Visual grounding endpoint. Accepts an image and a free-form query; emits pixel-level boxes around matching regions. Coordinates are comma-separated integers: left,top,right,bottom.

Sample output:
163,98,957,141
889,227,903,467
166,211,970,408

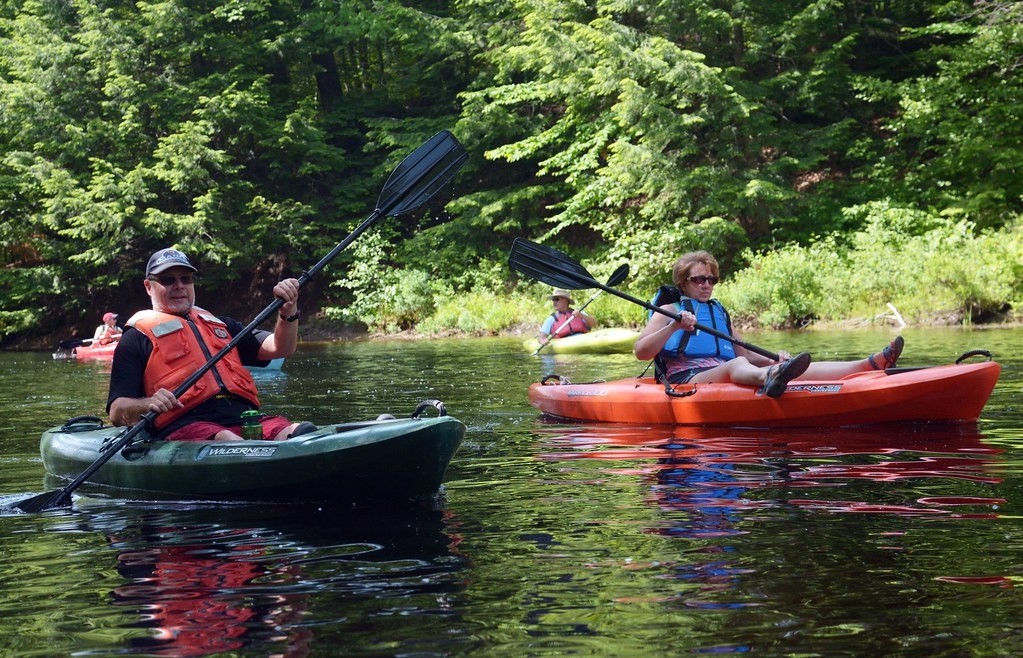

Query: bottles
240,410,263,440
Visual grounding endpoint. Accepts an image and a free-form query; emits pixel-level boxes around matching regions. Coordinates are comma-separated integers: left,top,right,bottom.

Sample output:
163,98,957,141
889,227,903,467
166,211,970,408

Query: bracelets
768,357,772,364
279,310,300,322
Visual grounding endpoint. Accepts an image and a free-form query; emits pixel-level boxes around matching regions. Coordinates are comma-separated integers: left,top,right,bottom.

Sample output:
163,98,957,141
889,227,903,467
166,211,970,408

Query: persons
92,313,122,348
538,288,597,346
635,250,904,398
106,249,396,441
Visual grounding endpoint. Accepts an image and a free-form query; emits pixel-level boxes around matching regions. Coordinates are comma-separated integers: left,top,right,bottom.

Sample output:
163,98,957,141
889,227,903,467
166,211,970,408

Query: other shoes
376,413,396,420
291,421,317,436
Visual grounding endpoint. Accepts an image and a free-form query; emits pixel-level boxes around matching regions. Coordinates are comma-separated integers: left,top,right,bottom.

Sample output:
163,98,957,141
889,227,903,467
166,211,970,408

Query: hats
103,313,118,322
547,290,574,305
146,249,197,277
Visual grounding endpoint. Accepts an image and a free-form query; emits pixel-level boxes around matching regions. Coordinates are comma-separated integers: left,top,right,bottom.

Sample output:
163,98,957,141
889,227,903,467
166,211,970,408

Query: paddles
9,129,471,509
59,333,122,350
529,262,631,356
506,235,779,362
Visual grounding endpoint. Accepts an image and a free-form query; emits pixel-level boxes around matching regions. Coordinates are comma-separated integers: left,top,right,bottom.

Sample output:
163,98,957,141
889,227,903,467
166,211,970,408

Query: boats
529,348,1002,441
38,400,470,504
73,340,124,361
523,325,642,355
244,357,286,373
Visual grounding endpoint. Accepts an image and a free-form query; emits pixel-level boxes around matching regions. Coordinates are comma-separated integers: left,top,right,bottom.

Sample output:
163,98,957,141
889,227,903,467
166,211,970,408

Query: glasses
552,297,567,301
147,275,195,285
685,276,718,284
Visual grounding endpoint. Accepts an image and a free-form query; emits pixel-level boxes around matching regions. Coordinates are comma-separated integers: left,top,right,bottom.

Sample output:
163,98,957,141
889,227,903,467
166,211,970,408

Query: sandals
869,336,903,370
764,353,810,398
